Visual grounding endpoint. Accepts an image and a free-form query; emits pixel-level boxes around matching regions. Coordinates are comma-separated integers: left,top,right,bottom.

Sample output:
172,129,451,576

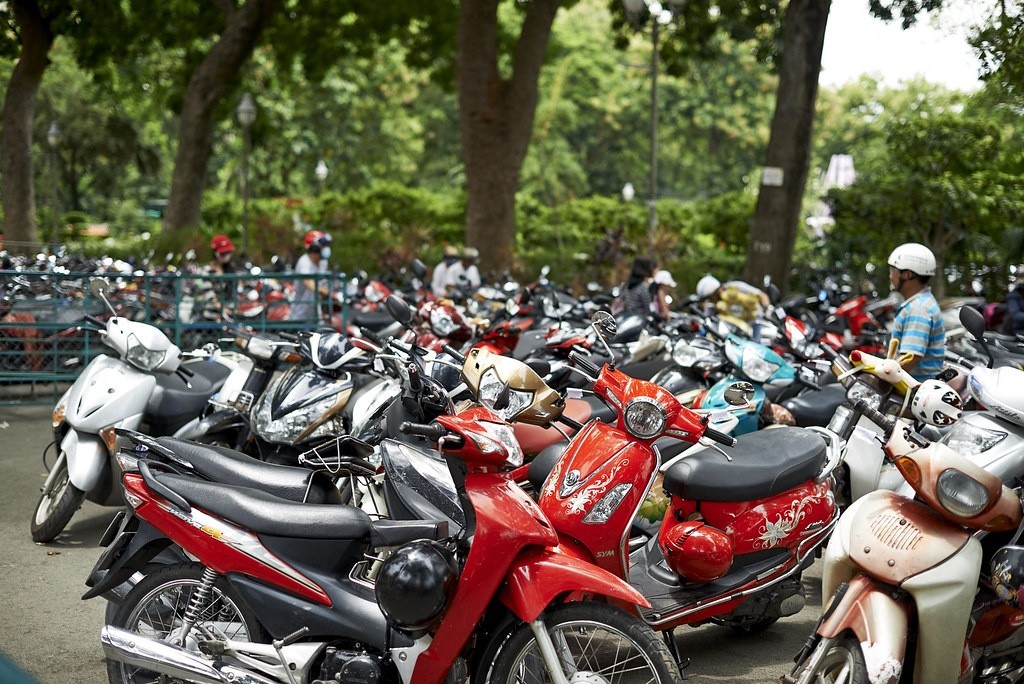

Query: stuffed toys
851,337,920,395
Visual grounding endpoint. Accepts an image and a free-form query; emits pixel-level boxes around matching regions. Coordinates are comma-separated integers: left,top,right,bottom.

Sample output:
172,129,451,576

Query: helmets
304,230,332,251
663,520,733,584
461,247,478,261
444,246,459,259
374,539,457,639
696,276,721,302
887,243,936,277
965,545,1024,648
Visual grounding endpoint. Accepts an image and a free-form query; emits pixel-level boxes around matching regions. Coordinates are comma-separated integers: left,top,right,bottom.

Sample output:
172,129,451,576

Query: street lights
235,91,258,245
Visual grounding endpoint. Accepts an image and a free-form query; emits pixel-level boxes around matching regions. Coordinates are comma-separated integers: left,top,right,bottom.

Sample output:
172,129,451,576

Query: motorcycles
0,243,1024,684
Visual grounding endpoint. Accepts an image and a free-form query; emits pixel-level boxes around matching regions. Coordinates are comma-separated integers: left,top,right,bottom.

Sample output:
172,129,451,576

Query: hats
211,235,235,254
655,270,677,288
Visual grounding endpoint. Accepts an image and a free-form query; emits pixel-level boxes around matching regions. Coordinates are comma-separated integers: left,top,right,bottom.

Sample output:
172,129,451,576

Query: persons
430,246,482,299
1000,281,1024,336
288,228,351,322
201,234,235,314
887,242,946,383
611,257,669,318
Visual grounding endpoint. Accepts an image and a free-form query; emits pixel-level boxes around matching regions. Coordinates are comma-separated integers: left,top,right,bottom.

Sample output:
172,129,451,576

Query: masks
218,253,232,263
321,247,331,259
